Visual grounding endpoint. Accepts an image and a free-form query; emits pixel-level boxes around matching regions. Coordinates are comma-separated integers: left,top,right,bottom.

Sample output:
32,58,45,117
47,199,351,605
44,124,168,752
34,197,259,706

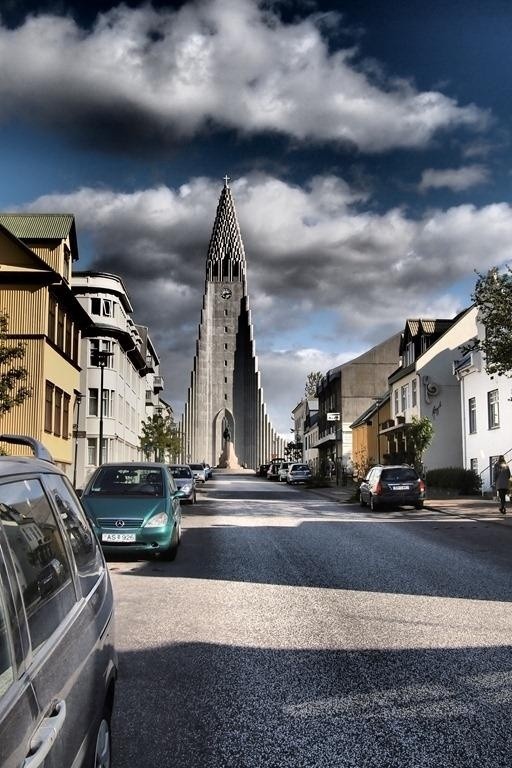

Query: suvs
356,461,427,513
0,430,122,768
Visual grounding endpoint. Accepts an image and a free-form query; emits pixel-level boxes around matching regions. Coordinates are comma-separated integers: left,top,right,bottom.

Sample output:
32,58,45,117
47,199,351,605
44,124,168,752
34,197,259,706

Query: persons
492,454,512,514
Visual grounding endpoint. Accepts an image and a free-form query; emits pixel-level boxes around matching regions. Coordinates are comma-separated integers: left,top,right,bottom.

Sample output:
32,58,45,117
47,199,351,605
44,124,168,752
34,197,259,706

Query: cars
73,459,219,564
259,456,312,485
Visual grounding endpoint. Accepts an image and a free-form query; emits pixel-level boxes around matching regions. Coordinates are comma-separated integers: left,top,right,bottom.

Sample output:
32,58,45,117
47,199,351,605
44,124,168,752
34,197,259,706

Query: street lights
371,394,384,468
89,348,115,470
